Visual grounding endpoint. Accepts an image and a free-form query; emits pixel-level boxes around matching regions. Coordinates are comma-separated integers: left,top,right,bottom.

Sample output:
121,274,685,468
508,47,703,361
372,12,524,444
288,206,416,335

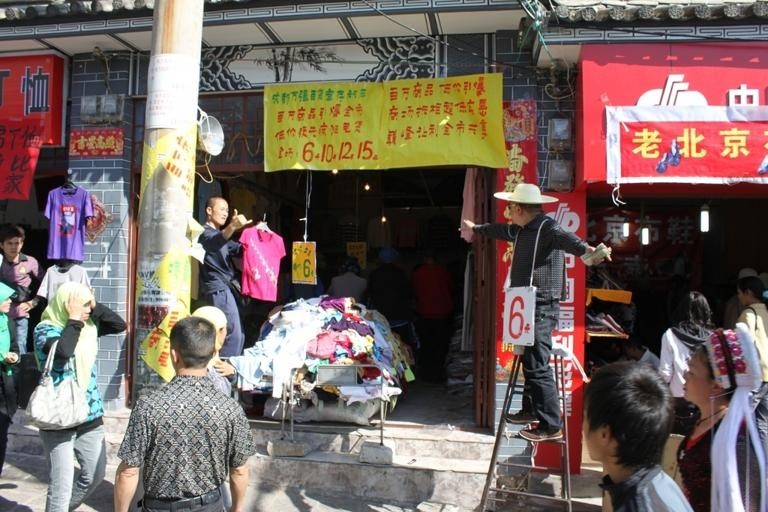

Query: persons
193,305,238,402
325,260,371,305
24,281,128,511
1,222,47,407
583,267,766,510
1,281,20,477
367,246,410,334
461,184,613,441
112,315,255,512
198,197,254,398
409,248,457,386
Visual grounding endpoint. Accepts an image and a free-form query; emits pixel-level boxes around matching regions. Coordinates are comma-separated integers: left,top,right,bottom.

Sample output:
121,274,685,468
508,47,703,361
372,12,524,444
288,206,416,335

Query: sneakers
519,430,563,442
507,412,540,423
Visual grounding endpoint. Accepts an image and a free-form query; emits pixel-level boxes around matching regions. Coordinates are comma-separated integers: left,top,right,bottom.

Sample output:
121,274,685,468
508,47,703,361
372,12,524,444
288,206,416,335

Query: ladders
479,344,570,512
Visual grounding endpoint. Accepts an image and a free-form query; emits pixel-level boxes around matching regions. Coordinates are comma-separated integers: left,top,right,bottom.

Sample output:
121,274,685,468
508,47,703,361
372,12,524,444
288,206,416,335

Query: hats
494,184,559,205
707,322,762,389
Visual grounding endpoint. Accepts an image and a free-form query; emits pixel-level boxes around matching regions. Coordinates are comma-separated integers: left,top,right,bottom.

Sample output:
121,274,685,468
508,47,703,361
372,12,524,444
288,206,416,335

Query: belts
138,489,220,509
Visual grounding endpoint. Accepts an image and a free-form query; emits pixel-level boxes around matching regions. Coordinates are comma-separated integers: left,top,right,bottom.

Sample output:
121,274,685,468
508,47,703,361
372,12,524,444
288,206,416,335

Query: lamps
622,200,710,246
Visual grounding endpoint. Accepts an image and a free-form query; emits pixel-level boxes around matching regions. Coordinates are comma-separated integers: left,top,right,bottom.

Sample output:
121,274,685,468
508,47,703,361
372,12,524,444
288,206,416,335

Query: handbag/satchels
669,397,701,435
8,284,32,302
25,340,89,430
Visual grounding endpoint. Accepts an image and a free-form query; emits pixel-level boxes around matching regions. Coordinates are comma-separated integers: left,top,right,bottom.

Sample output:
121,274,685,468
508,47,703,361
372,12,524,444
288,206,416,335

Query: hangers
59,173,78,196
255,212,273,235
53,258,74,271
